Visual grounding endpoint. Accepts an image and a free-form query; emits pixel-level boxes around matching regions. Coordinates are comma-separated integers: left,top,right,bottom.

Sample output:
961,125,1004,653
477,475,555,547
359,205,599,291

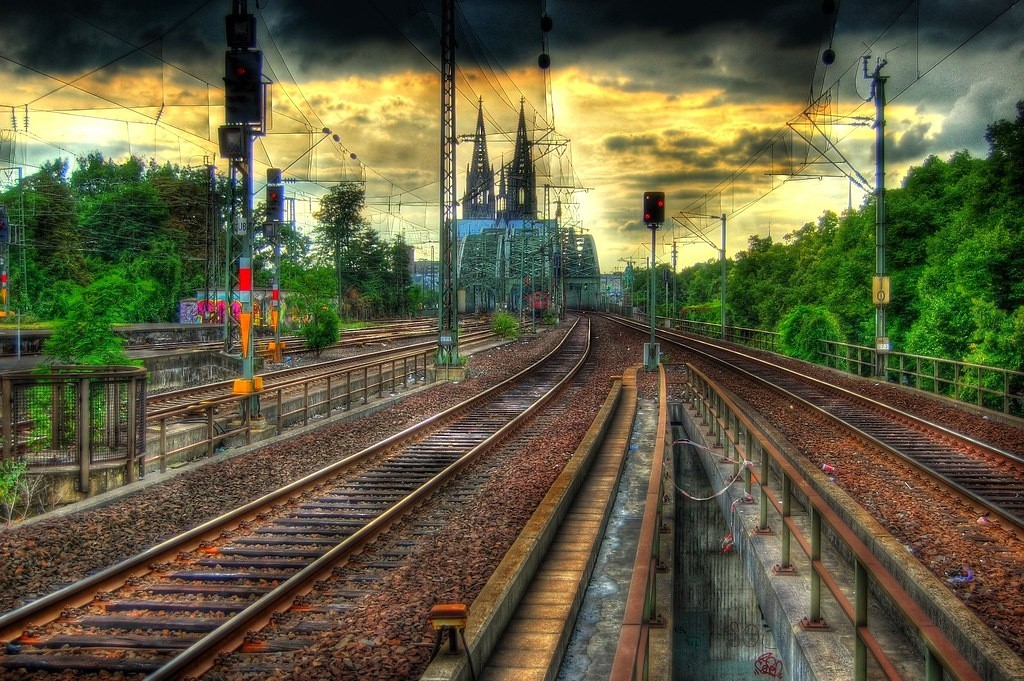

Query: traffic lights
643,191,665,223
263,222,276,237
266,184,285,222
0,217,9,241
218,126,245,156
225,48,265,128
